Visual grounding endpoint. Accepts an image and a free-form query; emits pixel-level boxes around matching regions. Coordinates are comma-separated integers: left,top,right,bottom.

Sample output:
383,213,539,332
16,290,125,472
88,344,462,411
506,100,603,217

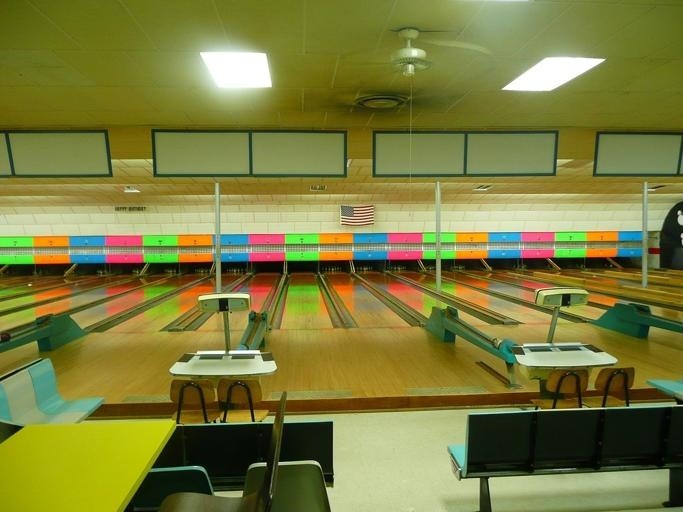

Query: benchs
447,405,683,511
174,421,335,489
0,357,104,426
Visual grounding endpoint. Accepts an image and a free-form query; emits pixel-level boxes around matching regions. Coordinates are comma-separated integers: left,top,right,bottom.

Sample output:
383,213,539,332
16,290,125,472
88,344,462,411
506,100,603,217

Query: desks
646,374,683,403
0,417,175,512
168,353,277,378
512,342,618,368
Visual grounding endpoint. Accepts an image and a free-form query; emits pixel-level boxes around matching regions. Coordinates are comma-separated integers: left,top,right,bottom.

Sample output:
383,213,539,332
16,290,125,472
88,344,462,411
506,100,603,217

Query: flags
340,205,374,226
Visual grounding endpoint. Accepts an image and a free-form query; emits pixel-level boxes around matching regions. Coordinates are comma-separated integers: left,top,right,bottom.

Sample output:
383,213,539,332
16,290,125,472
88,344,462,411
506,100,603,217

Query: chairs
242,460,332,511
217,379,269,421
531,369,588,407
595,369,634,407
134,465,213,511
170,379,222,423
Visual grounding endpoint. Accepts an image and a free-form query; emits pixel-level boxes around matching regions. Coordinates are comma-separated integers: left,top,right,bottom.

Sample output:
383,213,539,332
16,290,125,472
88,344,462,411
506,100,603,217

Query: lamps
401,65,418,77
354,95,407,111
